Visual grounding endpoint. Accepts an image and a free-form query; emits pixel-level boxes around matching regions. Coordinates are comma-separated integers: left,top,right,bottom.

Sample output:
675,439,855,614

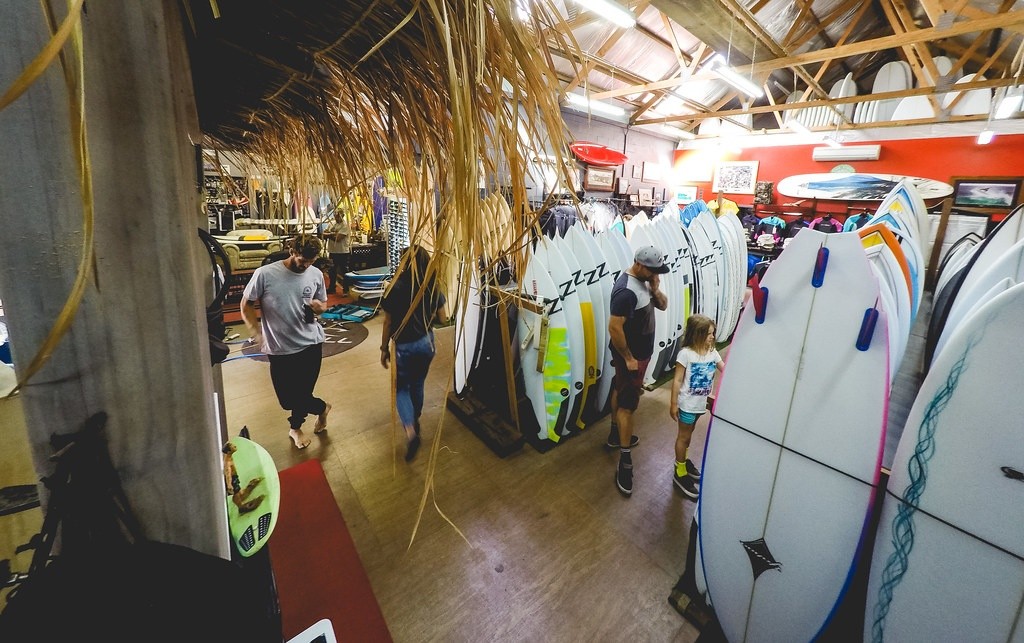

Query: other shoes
343,289,350,297
405,436,421,462
415,423,419,435
327,287,336,294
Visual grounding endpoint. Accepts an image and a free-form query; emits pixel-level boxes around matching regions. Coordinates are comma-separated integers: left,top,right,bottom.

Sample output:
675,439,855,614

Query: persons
608,245,670,495
380,245,447,462
240,234,332,450
322,208,350,298
670,315,726,499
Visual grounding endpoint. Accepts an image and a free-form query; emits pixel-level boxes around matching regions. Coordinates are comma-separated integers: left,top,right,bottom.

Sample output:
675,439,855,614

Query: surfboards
347,165,755,448
691,175,1024,643
224,436,282,557
254,166,404,237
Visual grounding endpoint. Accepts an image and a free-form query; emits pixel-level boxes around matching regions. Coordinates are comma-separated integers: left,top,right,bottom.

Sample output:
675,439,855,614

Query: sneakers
673,462,699,499
616,457,633,495
685,448,701,480
609,433,639,448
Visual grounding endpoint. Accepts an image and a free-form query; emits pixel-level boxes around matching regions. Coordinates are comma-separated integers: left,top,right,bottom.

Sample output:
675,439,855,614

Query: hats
634,245,670,273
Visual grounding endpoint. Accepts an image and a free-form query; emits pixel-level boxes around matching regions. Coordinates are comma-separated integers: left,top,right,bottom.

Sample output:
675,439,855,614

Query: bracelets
380,345,389,351
